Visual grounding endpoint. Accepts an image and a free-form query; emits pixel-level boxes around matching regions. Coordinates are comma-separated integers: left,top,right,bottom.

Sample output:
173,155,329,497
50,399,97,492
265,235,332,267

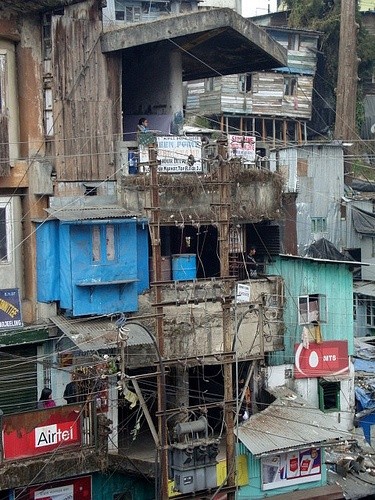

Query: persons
63,370,88,404
38,388,56,409
138,118,162,134
245,246,258,279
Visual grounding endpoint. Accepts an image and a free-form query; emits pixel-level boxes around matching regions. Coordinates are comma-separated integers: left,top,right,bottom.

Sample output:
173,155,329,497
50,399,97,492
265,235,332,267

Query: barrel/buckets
171,254,196,281
148,256,170,282
